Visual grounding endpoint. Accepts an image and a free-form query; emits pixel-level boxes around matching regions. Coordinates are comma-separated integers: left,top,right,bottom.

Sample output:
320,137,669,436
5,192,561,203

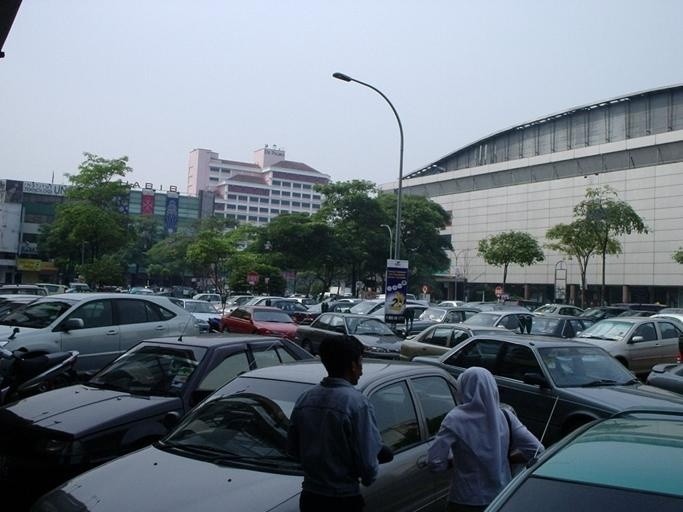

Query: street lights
333,70,408,293
379,219,405,259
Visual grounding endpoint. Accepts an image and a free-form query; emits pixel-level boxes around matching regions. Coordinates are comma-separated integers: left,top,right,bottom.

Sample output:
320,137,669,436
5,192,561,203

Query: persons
427,366,546,512
287,335,382,512
